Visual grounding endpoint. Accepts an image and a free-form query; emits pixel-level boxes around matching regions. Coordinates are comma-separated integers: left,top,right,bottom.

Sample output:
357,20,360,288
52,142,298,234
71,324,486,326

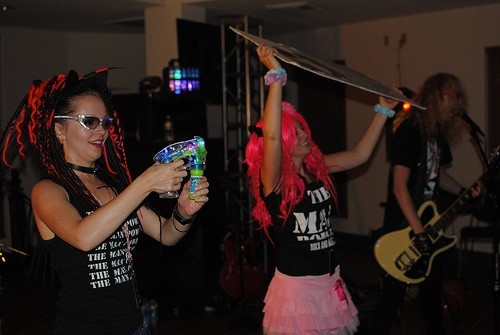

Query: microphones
457,109,485,136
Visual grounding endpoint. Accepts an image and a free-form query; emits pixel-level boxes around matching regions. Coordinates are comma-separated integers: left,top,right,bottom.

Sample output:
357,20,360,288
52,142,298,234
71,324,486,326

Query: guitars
373,148,500,284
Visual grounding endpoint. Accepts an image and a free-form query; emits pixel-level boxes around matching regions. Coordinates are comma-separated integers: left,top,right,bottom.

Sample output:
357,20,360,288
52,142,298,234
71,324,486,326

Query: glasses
54,115,114,130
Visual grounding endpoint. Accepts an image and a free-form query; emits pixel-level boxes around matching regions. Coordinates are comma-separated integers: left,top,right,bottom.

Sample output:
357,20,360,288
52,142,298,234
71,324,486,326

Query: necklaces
67,162,98,174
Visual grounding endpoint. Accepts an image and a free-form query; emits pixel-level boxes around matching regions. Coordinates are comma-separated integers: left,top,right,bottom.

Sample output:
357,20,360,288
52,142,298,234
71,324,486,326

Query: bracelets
173,204,196,233
263,68,287,86
373,104,395,118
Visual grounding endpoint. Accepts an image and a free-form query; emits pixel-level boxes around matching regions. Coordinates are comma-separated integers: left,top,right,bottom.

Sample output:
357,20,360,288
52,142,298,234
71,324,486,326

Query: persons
240,42,403,335
0,67,209,335
392,72,490,335
